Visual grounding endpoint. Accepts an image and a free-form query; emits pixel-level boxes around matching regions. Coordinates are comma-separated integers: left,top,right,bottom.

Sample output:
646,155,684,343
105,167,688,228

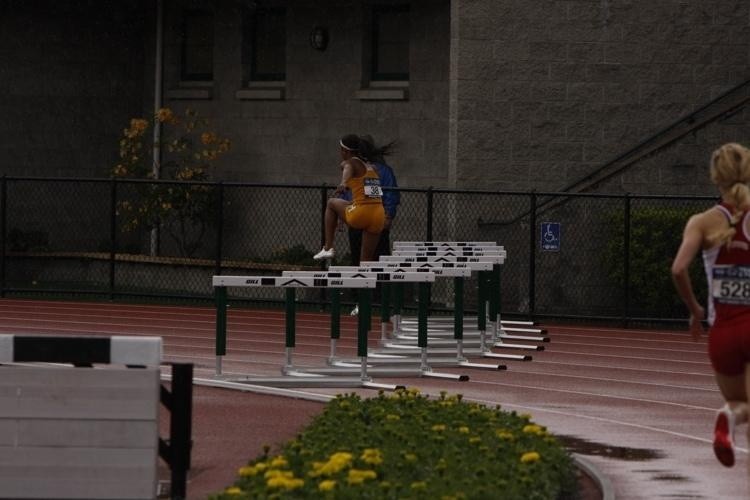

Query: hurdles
392,242,548,336
0,332,193,499
391,251,551,342
327,265,508,371
213,275,406,390
282,269,471,381
359,261,534,361
378,254,542,353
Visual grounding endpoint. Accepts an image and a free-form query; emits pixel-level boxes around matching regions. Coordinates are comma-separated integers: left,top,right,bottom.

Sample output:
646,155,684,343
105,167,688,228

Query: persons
671,142,750,467
312,133,387,318
344,135,401,316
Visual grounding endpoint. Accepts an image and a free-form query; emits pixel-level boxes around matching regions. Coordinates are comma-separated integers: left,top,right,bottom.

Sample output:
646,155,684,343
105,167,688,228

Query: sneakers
351,305,359,317
712,411,736,467
312,247,336,261
